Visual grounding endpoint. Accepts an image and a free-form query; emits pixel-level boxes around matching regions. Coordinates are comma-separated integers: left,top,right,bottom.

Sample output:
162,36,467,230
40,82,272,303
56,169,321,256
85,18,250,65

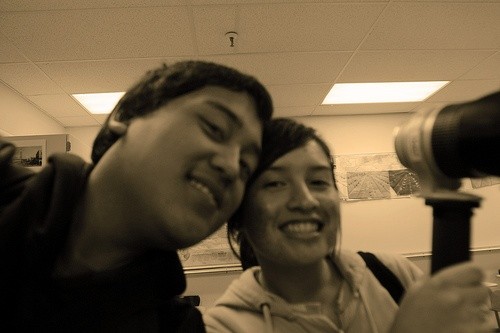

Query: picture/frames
177,221,243,274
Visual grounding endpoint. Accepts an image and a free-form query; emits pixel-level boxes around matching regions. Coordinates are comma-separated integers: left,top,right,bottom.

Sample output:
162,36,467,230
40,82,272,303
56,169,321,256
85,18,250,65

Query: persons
201,117,490,333
0,60,273,333
